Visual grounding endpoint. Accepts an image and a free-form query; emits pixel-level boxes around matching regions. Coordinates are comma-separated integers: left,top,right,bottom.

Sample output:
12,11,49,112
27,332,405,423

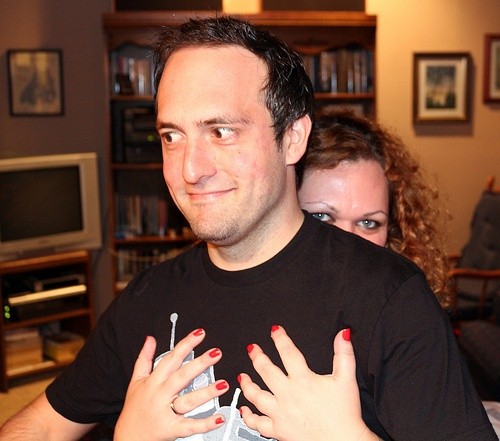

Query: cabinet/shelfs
104,10,378,294
0,249,96,393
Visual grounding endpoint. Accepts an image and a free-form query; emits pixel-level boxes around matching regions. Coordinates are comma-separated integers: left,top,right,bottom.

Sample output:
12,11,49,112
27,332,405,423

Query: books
117,247,164,280
113,194,169,236
303,45,371,95
108,52,156,97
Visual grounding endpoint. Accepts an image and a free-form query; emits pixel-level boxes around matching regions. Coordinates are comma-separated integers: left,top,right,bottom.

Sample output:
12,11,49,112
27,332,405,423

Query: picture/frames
483,32,500,102
413,50,471,122
9,47,63,116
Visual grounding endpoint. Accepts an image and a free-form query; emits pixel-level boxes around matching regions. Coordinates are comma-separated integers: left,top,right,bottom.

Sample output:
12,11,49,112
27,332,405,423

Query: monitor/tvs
0,152,102,262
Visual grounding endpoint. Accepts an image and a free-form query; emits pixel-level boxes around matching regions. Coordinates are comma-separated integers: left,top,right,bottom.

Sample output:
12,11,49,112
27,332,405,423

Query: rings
171,397,184,416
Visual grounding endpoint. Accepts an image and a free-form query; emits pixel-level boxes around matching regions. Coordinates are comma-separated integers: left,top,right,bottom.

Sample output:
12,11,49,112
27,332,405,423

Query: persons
113,110,458,441
0,10,500,441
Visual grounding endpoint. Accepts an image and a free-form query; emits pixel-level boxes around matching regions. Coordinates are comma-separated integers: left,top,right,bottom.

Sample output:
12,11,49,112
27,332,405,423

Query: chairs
442,175,500,388
